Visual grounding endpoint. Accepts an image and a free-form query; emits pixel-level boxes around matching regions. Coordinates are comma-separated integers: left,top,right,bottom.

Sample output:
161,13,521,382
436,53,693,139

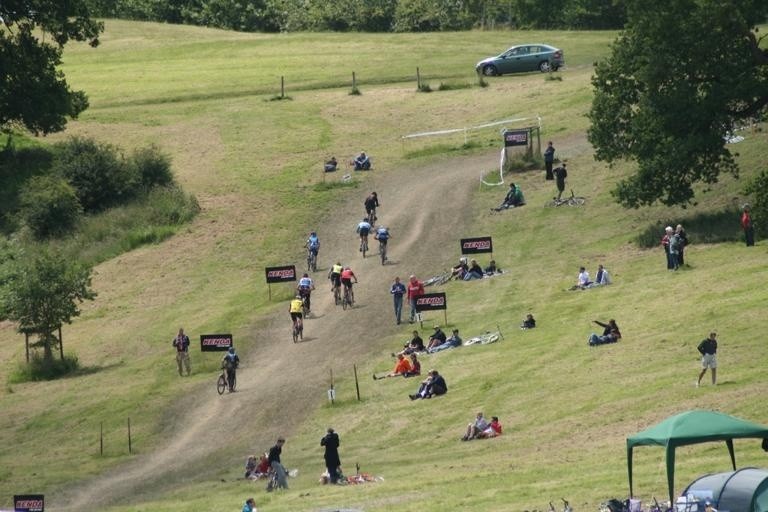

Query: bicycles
290,308,304,343
215,364,238,398
372,234,389,265
341,281,358,312
358,229,372,259
301,245,317,273
327,276,342,306
548,188,586,207
422,266,456,289
294,287,313,317
364,205,380,227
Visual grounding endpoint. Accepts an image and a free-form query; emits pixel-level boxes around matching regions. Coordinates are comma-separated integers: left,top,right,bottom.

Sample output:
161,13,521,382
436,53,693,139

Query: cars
471,43,566,79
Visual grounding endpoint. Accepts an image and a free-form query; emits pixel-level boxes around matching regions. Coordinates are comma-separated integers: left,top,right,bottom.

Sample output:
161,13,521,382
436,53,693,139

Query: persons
321,427,341,485
741,203,754,247
172,328,191,377
696,332,717,388
222,347,240,392
269,437,289,489
242,498,256,512
